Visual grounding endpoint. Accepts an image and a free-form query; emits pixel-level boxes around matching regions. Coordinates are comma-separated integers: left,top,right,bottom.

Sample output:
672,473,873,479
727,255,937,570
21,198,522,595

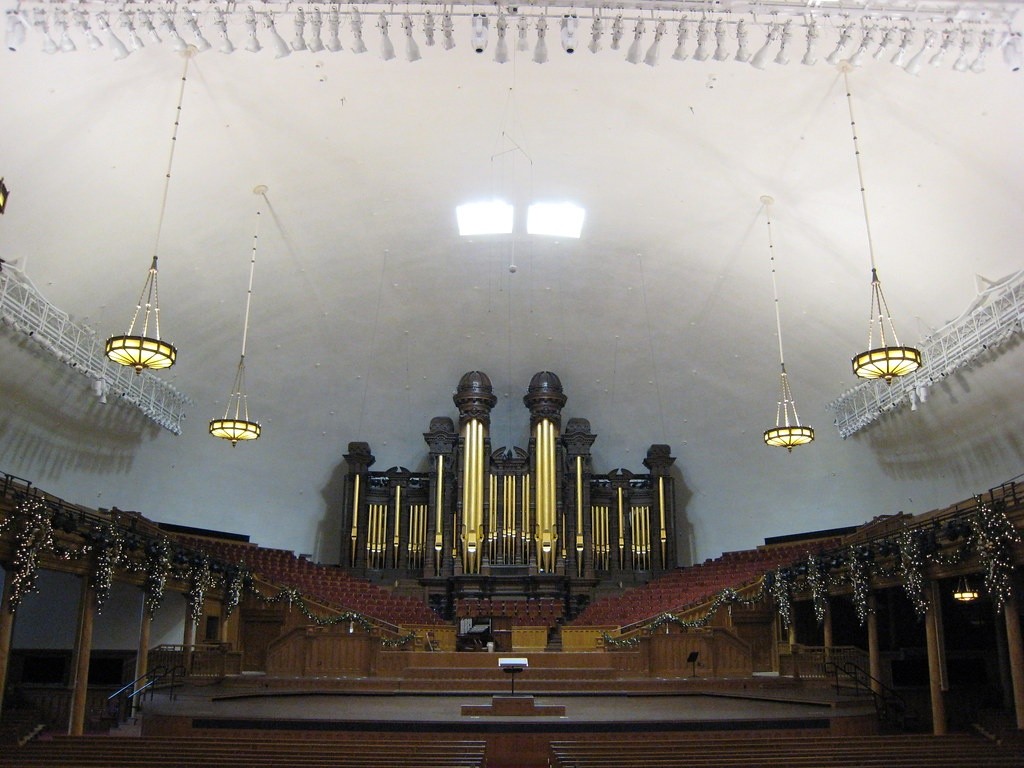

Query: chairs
492,600,502,608
540,599,562,607
529,607,539,616
570,537,842,629
517,599,527,607
480,609,490,616
505,600,514,606
493,608,502,616
456,600,466,608
505,607,515,616
468,600,478,608
512,616,556,627
175,535,445,626
470,608,478,617
529,598,539,607
541,605,550,616
480,600,490,608
517,607,527,616
552,606,562,617
456,607,467,617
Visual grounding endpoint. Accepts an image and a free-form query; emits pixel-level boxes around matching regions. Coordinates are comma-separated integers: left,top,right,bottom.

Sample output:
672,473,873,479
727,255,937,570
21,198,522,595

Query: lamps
953,577,979,602
516,16,530,52
493,12,512,64
761,195,813,452
625,4,1024,76
532,14,551,64
834,61,922,386
558,7,581,54
825,315,1024,439
610,8,625,50
8,6,367,62
103,45,198,376
587,8,604,54
400,11,422,62
0,307,195,437
374,10,396,61
423,10,437,46
440,5,457,50
209,185,268,445
471,12,490,54
